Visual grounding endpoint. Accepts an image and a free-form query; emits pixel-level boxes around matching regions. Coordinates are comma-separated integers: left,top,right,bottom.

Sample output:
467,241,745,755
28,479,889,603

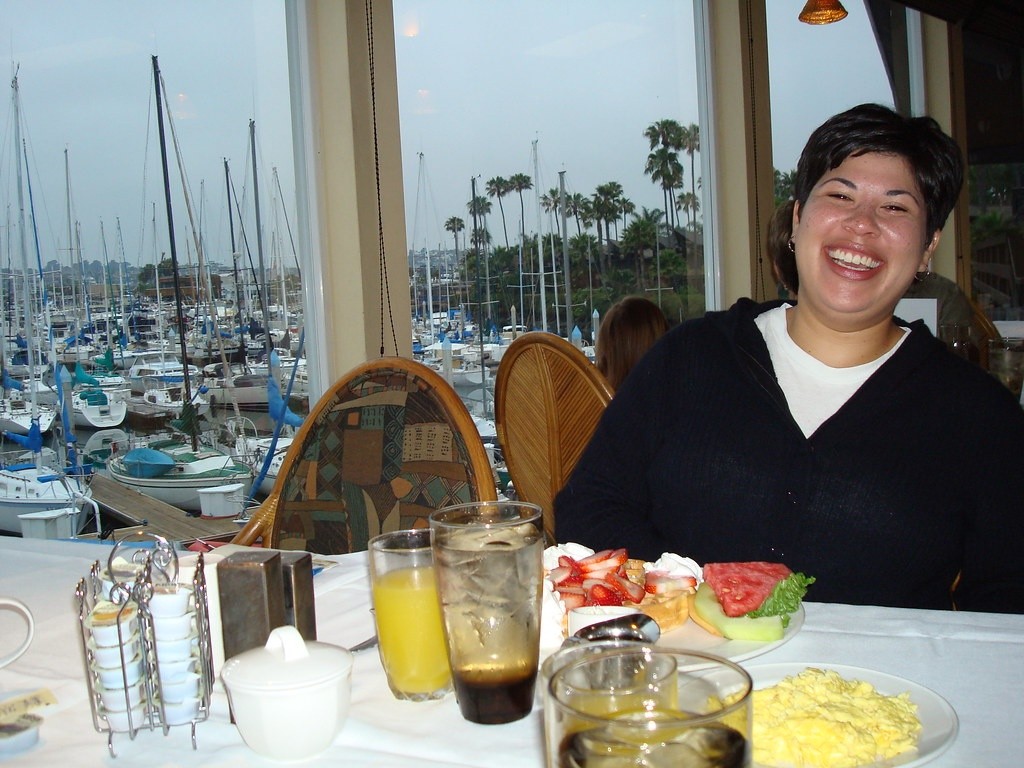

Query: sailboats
0,75,92,534
1,122,595,497
108,55,260,509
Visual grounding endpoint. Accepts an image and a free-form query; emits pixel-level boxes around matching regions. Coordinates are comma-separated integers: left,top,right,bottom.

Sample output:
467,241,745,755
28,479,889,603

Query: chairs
493,331,615,548
228,355,499,550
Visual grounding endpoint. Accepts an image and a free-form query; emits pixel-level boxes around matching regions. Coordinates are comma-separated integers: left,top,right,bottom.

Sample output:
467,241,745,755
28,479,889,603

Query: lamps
798,0,849,26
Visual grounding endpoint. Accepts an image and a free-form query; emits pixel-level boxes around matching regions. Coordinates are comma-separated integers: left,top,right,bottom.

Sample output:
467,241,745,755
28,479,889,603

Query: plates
540,585,804,675
737,664,960,767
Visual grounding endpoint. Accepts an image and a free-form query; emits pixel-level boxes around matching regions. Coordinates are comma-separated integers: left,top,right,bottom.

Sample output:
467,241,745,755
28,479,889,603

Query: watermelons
704,562,793,618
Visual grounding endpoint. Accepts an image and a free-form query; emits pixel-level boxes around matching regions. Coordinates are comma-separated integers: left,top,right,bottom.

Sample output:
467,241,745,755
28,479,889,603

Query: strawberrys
546,549,698,612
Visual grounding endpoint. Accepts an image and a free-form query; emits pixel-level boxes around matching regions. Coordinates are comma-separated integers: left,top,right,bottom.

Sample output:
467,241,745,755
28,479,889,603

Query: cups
429,501,543,724
541,640,753,768
987,337,1024,403
568,605,639,642
939,319,980,368
217,626,353,757
366,531,454,702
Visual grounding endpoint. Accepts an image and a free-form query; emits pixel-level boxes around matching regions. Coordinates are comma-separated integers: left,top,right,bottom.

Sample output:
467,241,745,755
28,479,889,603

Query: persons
595,297,671,393
768,197,800,299
530,103,1024,615
903,271,977,349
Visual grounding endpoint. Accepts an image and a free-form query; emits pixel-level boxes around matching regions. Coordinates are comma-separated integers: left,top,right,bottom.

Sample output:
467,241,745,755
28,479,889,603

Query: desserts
537,580,566,672
645,552,703,582
542,542,595,570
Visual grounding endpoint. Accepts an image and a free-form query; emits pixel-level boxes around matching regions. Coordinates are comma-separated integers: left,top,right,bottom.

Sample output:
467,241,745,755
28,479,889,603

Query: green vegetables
748,573,816,628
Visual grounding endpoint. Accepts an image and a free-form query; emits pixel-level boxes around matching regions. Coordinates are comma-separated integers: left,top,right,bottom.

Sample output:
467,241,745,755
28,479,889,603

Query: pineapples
690,584,784,639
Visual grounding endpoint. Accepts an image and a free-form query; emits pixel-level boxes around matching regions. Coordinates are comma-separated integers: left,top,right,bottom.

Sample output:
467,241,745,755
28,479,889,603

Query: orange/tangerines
623,591,689,633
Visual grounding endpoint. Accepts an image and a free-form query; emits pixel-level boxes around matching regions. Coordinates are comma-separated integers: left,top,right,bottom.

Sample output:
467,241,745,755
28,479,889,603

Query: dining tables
0,535,1024,768
